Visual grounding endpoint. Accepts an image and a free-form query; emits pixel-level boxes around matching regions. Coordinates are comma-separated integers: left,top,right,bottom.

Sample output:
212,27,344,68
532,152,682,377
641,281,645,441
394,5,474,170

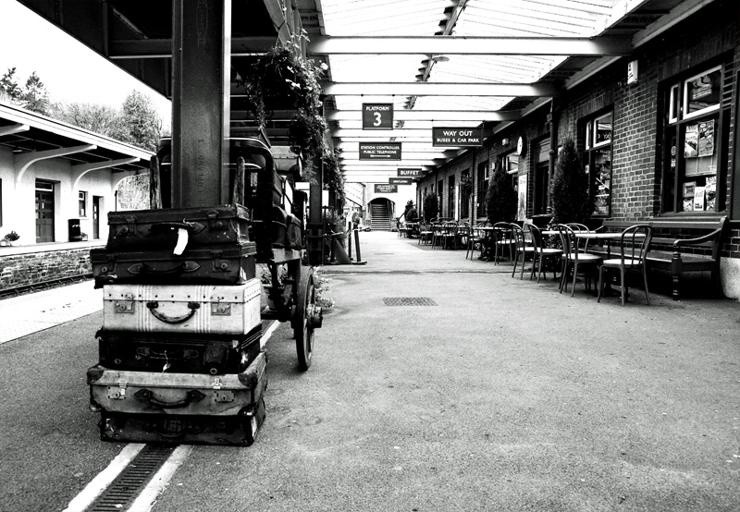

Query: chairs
395,215,653,306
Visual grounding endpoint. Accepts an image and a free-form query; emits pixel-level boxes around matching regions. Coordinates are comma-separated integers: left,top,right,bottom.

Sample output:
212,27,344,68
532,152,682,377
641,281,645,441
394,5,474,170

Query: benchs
597,209,729,302
